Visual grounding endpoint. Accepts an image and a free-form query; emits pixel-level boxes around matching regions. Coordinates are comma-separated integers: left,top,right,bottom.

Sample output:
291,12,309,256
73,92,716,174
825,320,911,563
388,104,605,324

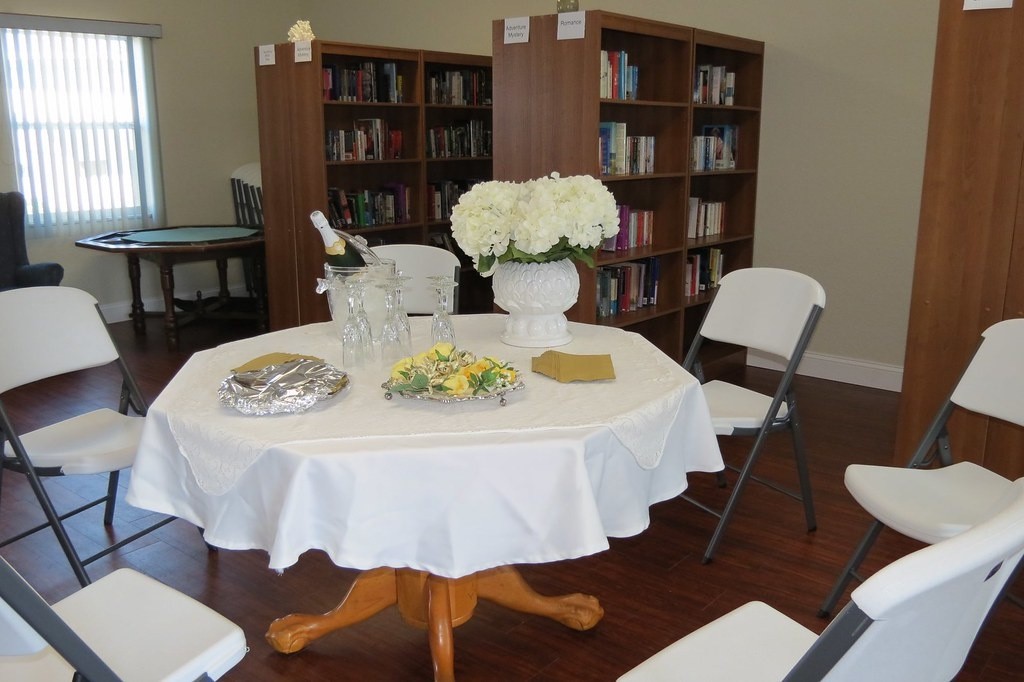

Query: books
429,115,493,158
692,123,738,171
327,182,411,229
688,195,725,238
685,247,724,296
596,257,660,318
597,121,656,175
430,178,477,220
323,58,403,104
599,47,637,101
325,117,403,161
600,203,654,251
429,231,454,256
694,62,736,106
430,67,492,105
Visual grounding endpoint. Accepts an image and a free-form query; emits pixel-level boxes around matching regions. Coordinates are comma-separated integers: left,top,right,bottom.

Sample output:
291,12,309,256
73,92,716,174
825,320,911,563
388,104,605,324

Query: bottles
310,211,366,268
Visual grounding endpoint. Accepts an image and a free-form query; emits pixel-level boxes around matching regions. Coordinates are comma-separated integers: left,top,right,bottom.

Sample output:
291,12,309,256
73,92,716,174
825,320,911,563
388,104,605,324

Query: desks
74,224,269,351
124,314,726,682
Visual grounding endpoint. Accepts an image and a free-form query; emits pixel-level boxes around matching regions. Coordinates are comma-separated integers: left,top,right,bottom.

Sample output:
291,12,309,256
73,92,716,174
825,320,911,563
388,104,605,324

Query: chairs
0,191,64,292
616,476,1024,682
0,285,218,588
0,556,250,682
361,245,461,316
816,317,1024,621
644,266,826,564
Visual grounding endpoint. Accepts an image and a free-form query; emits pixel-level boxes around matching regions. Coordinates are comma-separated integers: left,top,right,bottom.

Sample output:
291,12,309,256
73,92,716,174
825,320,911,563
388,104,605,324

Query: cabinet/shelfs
252,40,494,332
491,9,766,386
894,0,1024,481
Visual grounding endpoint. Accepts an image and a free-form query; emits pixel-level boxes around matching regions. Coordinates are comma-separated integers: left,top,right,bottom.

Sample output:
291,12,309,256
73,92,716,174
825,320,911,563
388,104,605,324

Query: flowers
384,342,516,398
449,171,620,279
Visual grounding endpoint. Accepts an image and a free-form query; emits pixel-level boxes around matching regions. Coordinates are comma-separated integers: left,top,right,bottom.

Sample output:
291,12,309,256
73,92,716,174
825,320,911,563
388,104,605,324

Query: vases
491,257,581,349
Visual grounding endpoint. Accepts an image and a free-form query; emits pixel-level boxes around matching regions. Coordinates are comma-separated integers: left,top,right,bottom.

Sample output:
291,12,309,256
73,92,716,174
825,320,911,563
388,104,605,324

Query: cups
316,258,396,345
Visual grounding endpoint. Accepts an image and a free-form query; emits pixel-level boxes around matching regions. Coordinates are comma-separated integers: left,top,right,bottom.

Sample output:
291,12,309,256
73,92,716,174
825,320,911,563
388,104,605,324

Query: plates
381,377,524,407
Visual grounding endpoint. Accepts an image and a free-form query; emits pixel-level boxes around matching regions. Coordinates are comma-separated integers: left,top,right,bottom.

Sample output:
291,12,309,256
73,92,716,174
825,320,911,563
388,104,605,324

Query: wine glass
340,274,457,368
